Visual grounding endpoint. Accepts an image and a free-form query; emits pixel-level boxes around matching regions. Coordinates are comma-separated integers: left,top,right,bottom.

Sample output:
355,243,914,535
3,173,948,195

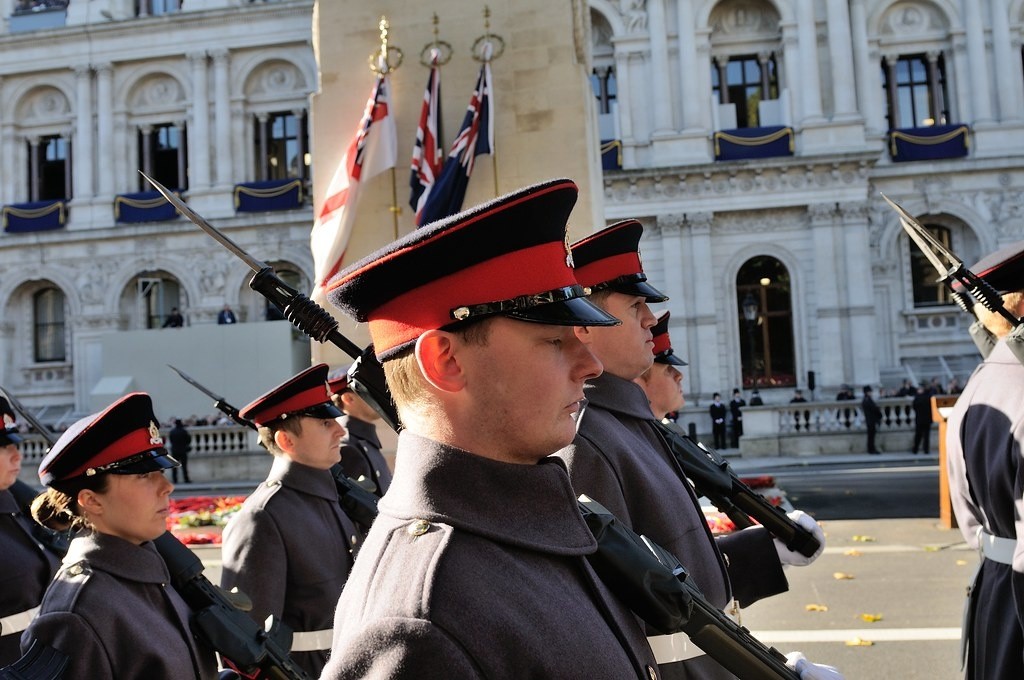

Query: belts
0,604,42,637
291,628,334,652
981,532,1017,565
646,596,742,664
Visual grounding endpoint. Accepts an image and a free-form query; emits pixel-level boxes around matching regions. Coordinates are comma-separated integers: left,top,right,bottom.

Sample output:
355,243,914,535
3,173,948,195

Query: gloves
772,510,825,567
716,418,723,424
784,651,844,680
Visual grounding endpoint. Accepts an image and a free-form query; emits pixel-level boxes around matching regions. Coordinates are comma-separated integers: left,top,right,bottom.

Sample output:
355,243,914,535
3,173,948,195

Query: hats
733,387,739,394
951,239,1024,304
238,363,347,429
840,383,850,390
649,309,688,366
752,389,760,394
38,393,182,487
863,385,873,393
324,178,624,364
919,379,929,386
326,365,354,395
569,218,670,304
713,392,720,399
0,396,25,447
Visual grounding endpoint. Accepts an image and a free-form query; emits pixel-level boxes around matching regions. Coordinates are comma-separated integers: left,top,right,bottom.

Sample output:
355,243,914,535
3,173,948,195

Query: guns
881,191,1024,328
688,477,758,530
166,362,382,518
899,215,979,322
135,168,801,680
0,385,316,680
655,419,820,558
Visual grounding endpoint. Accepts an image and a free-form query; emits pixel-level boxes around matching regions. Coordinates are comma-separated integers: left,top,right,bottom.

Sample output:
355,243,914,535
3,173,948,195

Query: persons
877,374,962,455
317,176,842,680
169,418,192,484
555,213,827,680
788,390,808,403
0,394,75,680
218,305,237,325
330,368,392,534
634,309,685,422
748,392,764,405
709,392,729,451
947,238,1024,680
218,362,365,680
162,309,184,328
861,386,883,457
834,385,859,429
19,392,249,680
730,388,747,449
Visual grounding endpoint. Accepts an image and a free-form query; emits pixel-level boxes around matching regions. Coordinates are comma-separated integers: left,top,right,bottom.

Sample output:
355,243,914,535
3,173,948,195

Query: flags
311,17,396,303
418,3,494,230
409,18,445,212
